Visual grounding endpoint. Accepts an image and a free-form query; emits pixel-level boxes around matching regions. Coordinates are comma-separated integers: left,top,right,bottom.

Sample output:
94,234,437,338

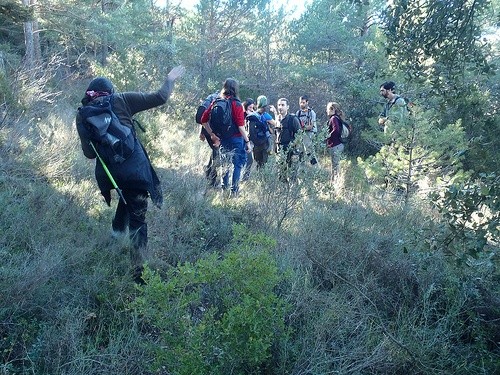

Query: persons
245,95,278,171
377,80,412,141
295,95,321,172
325,102,345,174
77,64,187,285
199,89,224,190
241,98,255,182
275,97,302,186
201,77,252,194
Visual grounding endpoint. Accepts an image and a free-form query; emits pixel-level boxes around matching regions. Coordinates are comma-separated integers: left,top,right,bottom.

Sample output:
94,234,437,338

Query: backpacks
196,93,220,124
209,96,240,139
331,114,353,143
246,113,272,146
76,94,137,166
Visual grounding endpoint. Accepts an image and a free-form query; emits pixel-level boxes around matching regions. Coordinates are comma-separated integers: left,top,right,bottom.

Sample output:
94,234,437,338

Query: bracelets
245,140,250,143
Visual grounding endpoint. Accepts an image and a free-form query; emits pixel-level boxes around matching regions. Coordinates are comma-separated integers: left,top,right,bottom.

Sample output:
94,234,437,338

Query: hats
257,95,267,107
